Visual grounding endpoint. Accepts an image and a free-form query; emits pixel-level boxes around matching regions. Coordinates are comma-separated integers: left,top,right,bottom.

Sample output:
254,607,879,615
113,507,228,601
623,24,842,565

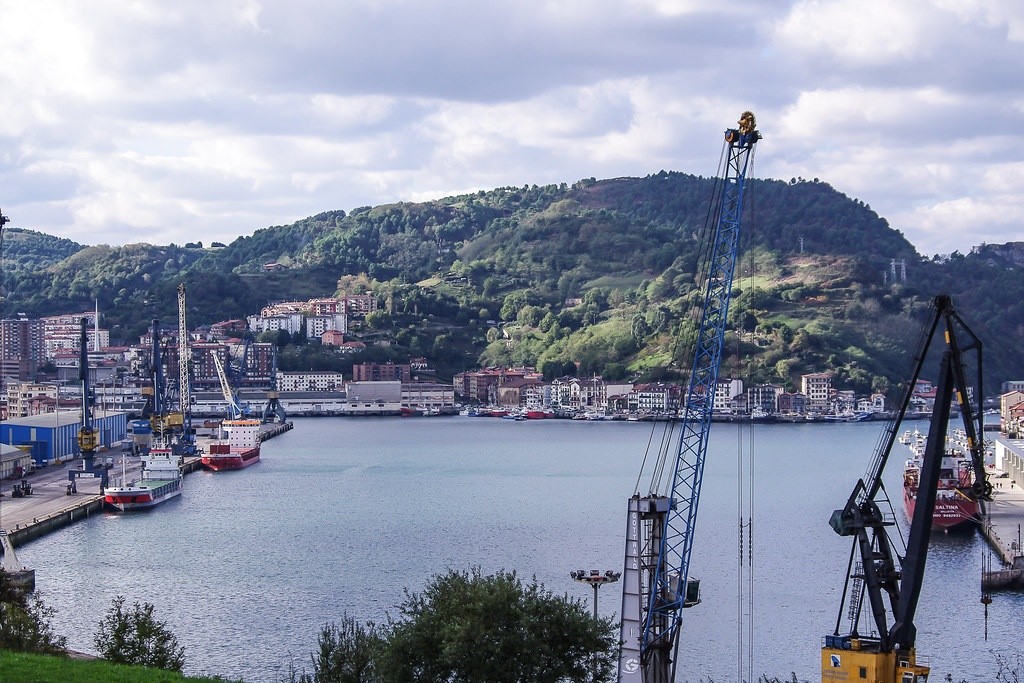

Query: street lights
569,569,622,683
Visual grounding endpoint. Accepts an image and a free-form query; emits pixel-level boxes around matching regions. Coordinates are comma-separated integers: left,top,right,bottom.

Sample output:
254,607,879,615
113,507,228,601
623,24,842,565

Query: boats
625,414,639,421
896,423,982,533
200,425,261,472
104,420,184,512
484,393,614,420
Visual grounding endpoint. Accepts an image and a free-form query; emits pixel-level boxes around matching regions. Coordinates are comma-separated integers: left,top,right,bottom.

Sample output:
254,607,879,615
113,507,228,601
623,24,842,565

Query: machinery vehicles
815,291,999,681
614,110,764,683
211,352,262,427
65,317,114,498
147,283,199,458
